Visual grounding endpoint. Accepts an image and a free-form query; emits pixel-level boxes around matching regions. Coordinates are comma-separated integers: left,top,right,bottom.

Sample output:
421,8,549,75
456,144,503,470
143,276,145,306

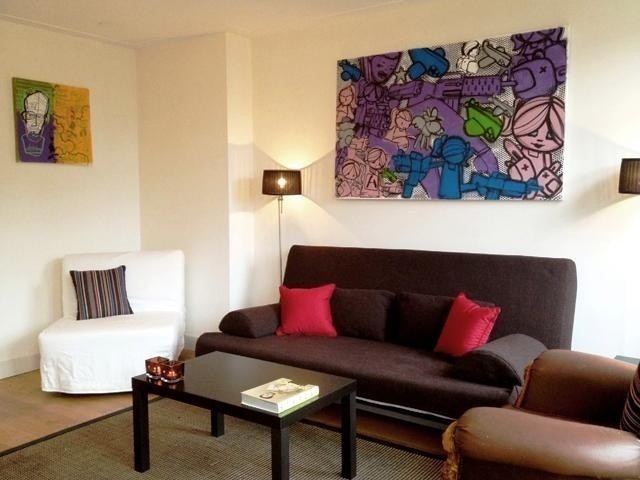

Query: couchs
196,245,577,431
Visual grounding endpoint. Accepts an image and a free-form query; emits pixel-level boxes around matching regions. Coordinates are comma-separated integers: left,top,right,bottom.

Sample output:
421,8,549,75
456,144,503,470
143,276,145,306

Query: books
239,376,321,415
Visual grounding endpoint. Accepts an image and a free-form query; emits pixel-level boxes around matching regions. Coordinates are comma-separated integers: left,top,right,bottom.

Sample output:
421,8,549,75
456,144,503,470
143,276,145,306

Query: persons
258,379,310,400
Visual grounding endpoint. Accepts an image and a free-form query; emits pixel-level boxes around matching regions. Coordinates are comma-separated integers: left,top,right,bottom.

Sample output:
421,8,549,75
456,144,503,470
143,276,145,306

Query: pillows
70,265,134,320
618,363,640,439
276,285,501,358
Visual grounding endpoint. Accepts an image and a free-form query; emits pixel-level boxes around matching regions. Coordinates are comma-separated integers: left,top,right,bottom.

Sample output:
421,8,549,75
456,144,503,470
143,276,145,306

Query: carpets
0,396,448,480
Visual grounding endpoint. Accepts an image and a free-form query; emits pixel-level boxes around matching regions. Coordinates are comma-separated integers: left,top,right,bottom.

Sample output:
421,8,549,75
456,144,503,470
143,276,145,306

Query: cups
145,355,185,385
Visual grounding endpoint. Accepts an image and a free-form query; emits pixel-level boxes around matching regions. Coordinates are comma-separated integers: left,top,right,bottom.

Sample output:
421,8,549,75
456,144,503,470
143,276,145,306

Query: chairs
38,249,186,395
442,349,640,480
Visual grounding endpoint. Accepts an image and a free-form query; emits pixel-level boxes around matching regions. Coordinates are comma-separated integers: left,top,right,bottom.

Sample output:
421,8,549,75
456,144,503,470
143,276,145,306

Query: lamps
619,159,640,193
262,170,301,284
